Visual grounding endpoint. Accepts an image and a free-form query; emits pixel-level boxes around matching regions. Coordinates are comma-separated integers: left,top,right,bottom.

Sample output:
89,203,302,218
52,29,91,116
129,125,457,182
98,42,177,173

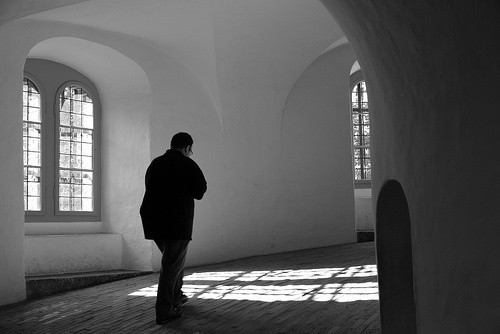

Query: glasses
189,148,193,155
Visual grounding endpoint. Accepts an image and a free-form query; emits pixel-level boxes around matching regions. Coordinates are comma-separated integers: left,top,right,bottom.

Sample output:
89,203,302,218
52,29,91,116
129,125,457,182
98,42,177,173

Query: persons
140,132,209,325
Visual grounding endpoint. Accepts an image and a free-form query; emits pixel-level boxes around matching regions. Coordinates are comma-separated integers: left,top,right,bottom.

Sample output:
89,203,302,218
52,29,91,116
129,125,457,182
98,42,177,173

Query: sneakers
157,308,182,325
174,295,188,307
